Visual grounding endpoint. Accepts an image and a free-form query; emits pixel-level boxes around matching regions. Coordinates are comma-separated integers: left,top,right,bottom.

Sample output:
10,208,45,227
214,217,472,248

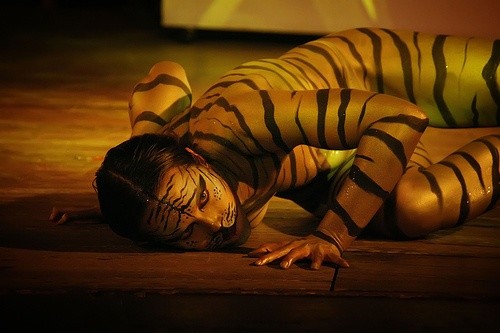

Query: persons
48,28,500,272
